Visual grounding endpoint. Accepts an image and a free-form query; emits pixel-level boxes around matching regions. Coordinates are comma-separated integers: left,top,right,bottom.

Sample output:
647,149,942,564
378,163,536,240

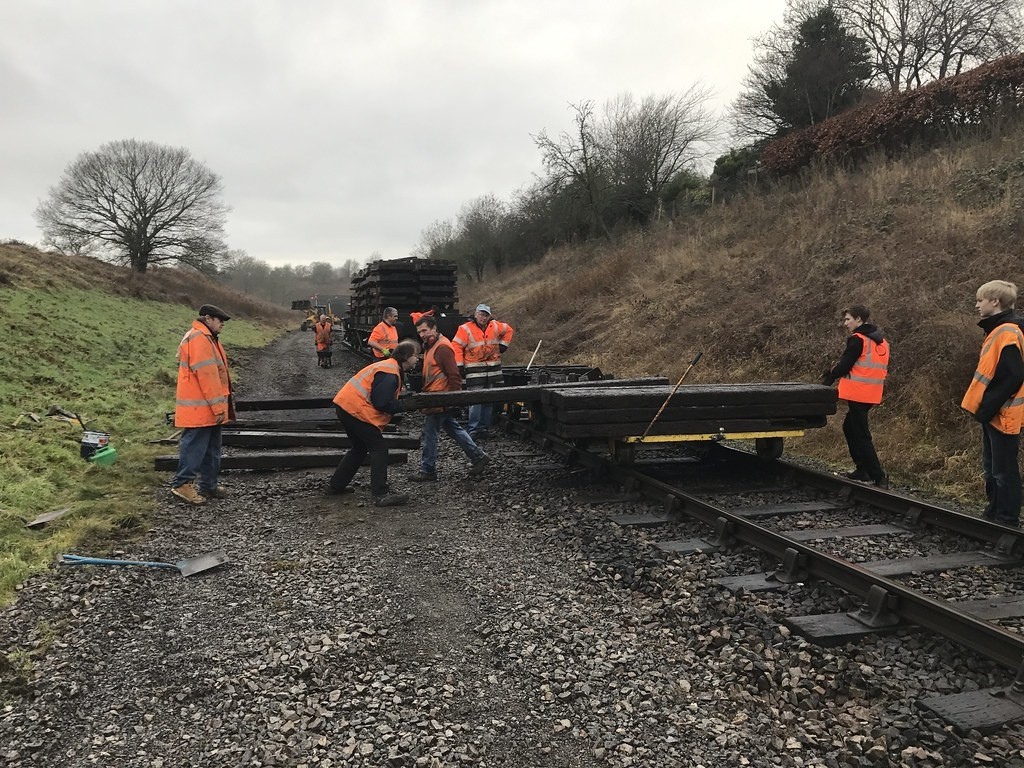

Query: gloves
500,344,507,353
457,366,466,378
382,348,390,355
821,371,836,386
403,396,425,410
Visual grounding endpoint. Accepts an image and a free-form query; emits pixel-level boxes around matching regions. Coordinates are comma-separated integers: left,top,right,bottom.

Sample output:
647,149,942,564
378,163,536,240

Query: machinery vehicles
290,299,340,332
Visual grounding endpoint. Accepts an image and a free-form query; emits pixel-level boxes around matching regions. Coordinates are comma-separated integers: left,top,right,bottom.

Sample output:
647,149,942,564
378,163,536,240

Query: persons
314,314,333,362
452,303,514,439
368,306,399,363
958,279,1024,526
407,315,491,482
326,337,422,507
830,305,890,489
410,305,435,353
334,315,344,325
170,304,237,504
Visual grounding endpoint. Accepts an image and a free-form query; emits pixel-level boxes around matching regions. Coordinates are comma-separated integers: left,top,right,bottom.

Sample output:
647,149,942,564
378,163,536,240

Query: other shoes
480,431,496,439
846,471,869,481
875,472,885,486
470,431,476,441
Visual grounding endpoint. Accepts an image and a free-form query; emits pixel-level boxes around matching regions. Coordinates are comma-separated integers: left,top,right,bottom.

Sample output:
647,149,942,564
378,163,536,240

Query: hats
198,304,230,321
476,303,491,315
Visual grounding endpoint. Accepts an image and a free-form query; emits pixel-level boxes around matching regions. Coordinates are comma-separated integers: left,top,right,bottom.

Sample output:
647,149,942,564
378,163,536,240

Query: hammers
148,431,181,444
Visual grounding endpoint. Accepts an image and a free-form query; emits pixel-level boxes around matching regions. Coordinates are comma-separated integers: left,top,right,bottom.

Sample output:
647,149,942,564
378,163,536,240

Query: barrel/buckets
81,431,111,462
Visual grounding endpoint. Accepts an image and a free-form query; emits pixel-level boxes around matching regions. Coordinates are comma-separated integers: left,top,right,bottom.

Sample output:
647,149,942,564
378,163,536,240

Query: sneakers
408,471,437,481
326,486,355,494
171,483,206,503
376,493,408,506
469,454,491,476
201,486,231,497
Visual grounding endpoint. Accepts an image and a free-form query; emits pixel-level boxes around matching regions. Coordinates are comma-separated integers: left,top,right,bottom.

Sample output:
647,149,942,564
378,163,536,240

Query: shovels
56,550,229,578
0,508,70,527
